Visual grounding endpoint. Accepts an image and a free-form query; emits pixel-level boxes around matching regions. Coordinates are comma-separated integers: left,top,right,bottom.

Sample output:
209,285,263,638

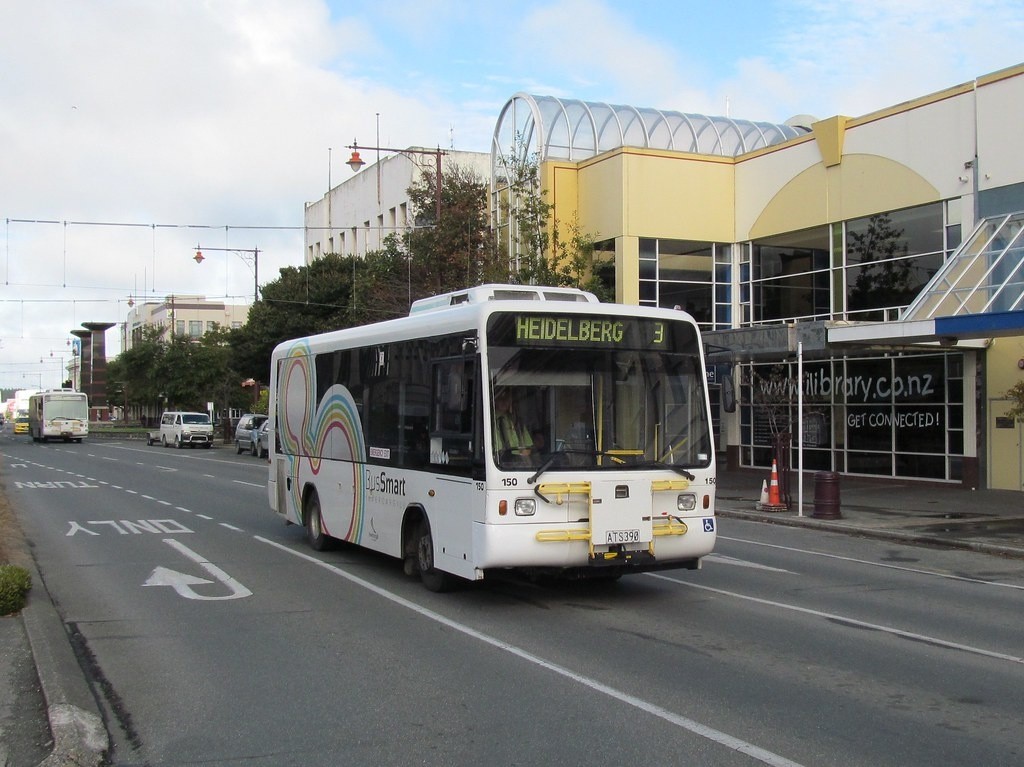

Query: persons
493,390,533,455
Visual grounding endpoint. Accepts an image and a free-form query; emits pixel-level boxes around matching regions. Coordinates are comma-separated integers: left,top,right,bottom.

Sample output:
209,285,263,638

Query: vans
160,411,213,449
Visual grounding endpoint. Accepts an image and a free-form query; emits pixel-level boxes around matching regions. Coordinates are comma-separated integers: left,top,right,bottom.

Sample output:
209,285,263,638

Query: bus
28,390,88,443
265,286,718,595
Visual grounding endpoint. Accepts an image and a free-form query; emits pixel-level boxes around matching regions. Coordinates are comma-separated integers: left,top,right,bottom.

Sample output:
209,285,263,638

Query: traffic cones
755,459,787,511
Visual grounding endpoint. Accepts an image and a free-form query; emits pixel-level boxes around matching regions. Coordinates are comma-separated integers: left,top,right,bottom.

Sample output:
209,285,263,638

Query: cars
234,413,268,457
14,417,29,433
